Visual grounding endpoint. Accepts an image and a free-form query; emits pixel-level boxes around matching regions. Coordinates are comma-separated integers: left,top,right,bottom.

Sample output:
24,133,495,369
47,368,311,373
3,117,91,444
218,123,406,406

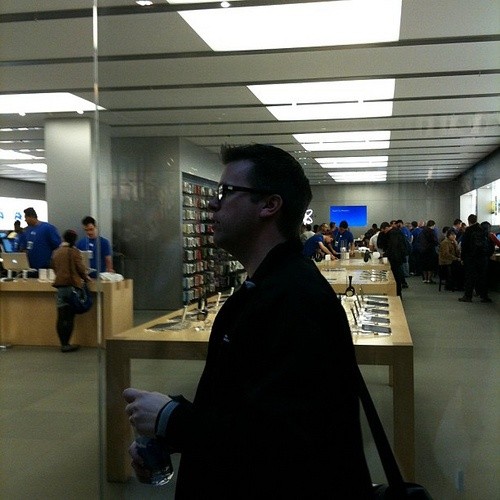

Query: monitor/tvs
2,252,30,270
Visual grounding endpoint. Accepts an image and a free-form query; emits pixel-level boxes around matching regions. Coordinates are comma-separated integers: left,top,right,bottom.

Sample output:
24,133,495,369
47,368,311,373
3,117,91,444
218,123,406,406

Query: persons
300,213,500,300
49,230,92,353
458,214,492,303
4,221,24,251
76,216,114,279
16,207,62,278
122,141,374,500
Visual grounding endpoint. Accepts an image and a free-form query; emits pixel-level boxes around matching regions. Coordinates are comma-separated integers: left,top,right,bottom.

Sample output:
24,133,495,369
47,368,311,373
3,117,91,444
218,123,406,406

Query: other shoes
480,298,492,302
427,280,431,283
423,280,427,282
458,296,472,302
62,346,78,352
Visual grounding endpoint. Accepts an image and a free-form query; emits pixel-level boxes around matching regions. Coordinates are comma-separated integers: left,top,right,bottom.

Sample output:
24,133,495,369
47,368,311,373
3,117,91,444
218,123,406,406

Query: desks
0,246,421,500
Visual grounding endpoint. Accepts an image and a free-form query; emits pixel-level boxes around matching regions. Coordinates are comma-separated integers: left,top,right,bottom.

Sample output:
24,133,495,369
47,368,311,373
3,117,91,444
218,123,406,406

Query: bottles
135,426,174,486
345,276,355,296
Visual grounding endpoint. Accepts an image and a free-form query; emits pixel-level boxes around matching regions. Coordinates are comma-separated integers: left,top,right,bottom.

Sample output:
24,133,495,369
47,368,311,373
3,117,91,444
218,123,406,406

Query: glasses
217,183,253,200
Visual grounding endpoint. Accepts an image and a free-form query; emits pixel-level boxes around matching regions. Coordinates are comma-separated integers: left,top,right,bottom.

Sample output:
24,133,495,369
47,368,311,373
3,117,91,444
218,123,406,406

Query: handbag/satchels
63,283,93,314
372,482,433,500
